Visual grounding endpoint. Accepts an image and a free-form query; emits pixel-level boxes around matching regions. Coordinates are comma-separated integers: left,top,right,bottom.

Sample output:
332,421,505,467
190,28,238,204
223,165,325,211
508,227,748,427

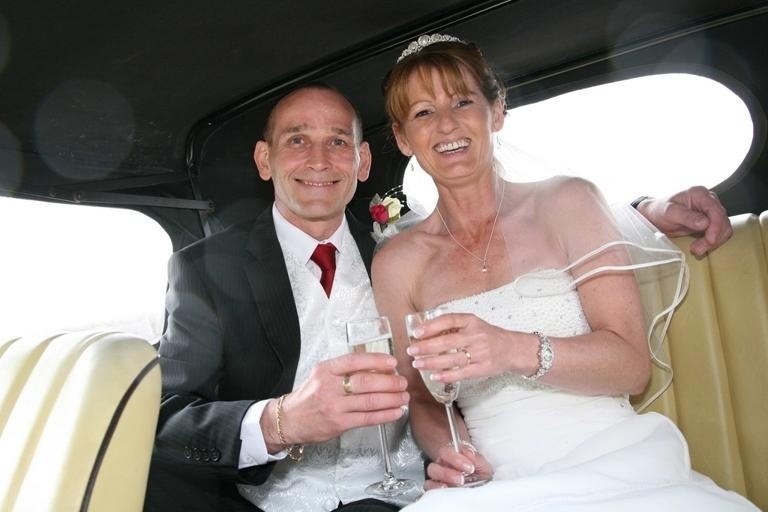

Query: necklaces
435,180,504,272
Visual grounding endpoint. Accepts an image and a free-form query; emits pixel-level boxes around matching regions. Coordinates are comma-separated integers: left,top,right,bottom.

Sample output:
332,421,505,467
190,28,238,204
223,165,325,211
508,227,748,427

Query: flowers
368,182,409,225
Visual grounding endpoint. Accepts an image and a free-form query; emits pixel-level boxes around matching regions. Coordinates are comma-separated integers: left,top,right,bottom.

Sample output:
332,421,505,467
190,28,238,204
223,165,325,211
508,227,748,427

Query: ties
310,243,336,299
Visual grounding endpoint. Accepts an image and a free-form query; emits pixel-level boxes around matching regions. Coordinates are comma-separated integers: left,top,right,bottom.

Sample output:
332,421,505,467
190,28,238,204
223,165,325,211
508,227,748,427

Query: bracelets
276,394,304,462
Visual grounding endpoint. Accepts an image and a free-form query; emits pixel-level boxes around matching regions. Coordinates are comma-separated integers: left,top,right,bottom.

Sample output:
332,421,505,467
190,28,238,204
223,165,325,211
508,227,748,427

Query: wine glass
405,306,491,490
344,316,416,498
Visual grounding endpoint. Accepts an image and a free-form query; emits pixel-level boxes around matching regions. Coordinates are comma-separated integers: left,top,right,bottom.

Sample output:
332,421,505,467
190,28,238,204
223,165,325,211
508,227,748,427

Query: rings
464,351,471,366
343,377,351,394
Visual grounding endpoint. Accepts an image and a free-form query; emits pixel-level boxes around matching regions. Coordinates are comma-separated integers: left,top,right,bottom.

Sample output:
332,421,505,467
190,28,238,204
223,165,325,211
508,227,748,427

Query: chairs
629,207,768,511
0,324,166,512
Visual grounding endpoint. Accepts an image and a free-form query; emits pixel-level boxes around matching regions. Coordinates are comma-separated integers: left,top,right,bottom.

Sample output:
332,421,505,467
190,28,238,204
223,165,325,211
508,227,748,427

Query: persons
370,33,763,512
154,82,733,512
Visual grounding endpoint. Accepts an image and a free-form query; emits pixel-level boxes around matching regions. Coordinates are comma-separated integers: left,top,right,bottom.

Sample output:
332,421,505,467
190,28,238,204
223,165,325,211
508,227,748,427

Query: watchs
521,331,553,381
